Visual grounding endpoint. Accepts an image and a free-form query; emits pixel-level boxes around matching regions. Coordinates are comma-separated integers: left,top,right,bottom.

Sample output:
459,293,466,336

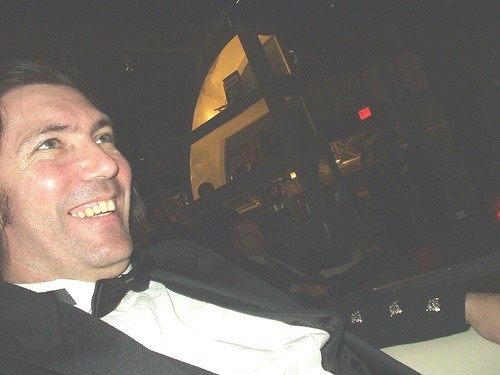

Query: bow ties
91,259,150,319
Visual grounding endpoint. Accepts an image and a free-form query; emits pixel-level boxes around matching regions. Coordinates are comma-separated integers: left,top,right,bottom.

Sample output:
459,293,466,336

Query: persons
1,59,500,375
129,147,452,299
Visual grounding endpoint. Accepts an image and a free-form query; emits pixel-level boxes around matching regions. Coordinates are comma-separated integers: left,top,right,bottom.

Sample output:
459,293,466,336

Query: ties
258,253,305,282
